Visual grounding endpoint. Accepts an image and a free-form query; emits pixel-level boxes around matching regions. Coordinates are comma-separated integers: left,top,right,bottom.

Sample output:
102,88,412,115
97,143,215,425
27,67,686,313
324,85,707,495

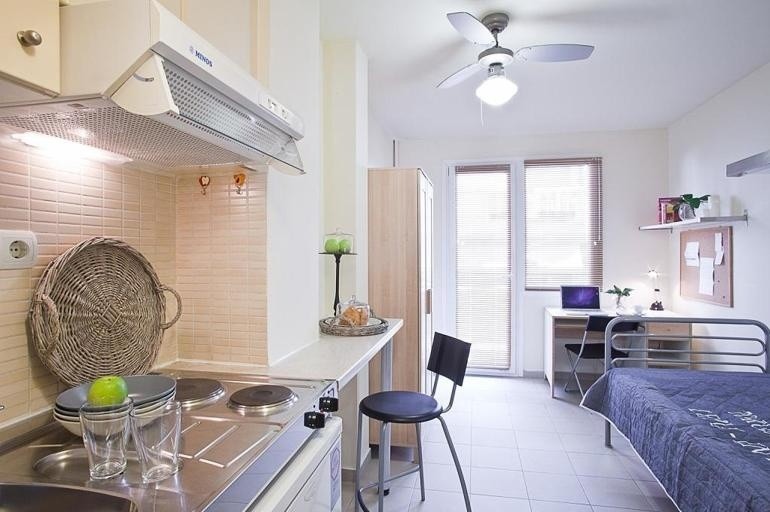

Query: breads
340,307,369,325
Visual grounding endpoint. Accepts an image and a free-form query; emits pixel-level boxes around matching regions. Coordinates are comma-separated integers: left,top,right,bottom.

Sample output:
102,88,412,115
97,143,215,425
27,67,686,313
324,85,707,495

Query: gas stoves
148,366,340,433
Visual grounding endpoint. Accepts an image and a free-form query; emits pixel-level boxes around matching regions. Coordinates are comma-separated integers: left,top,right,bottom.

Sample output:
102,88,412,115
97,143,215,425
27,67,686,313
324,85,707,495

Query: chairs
355,330,473,512
564,315,640,397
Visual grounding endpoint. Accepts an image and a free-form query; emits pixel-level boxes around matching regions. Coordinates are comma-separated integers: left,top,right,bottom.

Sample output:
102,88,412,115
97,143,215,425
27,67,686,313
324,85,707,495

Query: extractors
55,0,310,180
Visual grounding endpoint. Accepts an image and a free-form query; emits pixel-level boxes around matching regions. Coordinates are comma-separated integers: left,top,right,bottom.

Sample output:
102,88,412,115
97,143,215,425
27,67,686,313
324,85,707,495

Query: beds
600,315,769,511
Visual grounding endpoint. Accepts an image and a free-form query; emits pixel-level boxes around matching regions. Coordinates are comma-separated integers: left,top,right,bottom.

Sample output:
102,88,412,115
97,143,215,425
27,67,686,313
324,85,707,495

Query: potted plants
604,285,636,309
671,194,710,221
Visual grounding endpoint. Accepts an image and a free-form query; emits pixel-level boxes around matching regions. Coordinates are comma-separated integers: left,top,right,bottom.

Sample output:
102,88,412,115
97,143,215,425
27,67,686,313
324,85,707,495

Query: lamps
474,62,518,108
649,269,663,310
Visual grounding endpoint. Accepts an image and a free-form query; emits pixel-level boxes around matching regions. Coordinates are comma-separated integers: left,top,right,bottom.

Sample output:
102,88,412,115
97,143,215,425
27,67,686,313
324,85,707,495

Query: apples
325,239,340,253
86,375,129,406
338,240,351,253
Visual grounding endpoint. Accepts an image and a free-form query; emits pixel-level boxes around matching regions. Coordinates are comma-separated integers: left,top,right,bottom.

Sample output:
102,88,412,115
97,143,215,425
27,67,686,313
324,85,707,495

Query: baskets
26,234,184,392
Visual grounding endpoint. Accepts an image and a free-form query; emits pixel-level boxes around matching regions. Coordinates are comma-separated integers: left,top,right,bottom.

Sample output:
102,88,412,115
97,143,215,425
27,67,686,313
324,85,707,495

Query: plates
52,376,177,440
323,316,383,328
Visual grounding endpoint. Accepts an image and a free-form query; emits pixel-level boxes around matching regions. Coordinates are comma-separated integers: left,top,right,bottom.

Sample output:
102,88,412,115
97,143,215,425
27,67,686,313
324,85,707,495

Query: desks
544,307,692,398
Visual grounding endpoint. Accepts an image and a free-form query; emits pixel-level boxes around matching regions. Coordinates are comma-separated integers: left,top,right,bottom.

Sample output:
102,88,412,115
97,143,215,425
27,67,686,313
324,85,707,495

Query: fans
435,11,594,91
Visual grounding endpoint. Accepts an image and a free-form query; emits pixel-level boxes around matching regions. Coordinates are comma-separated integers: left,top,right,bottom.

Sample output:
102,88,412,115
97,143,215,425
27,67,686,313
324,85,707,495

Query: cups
80,398,180,484
335,295,370,326
707,195,720,216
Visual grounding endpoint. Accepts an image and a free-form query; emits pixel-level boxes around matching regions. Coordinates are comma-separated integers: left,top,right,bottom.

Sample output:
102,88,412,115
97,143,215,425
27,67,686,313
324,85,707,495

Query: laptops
560,285,609,316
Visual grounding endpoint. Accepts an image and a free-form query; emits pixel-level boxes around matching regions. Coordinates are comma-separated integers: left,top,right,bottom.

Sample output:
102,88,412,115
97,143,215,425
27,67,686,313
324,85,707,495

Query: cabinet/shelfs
368,166,433,463
1,2,62,106
